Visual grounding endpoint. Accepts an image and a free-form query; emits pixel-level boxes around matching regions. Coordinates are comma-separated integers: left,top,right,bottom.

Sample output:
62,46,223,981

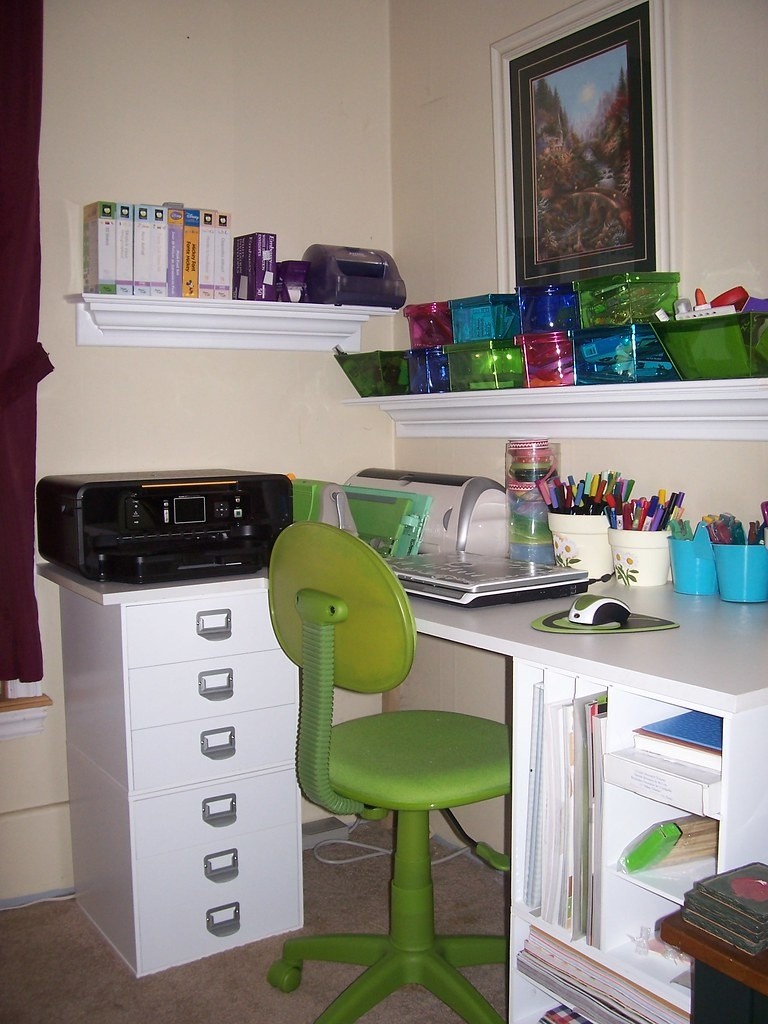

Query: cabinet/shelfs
58,591,305,979
508,666,768,1024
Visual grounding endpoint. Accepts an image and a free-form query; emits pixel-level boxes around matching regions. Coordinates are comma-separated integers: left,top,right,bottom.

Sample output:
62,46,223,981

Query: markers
534,469,636,516
668,499,768,548
602,489,686,532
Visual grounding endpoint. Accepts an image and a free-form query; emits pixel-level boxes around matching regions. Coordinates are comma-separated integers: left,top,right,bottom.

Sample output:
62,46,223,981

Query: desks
661,909,768,1024
38,566,768,713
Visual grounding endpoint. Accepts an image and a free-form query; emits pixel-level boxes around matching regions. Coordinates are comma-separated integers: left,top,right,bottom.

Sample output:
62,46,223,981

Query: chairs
266,520,513,1024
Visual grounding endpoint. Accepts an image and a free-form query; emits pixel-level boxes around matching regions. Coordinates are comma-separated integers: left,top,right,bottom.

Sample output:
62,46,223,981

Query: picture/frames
489,0,683,294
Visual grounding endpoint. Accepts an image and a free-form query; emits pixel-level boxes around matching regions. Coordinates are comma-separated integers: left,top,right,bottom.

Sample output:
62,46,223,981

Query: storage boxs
505,443,560,566
333,272,768,398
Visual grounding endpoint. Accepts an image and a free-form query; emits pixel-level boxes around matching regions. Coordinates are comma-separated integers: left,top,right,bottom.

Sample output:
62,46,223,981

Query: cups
547,512,616,583
608,527,669,587
712,543,768,603
667,524,719,596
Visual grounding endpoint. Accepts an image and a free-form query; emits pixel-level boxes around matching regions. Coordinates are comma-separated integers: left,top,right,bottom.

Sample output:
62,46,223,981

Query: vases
547,512,615,579
711,544,768,602
666,521,718,596
608,528,671,586
